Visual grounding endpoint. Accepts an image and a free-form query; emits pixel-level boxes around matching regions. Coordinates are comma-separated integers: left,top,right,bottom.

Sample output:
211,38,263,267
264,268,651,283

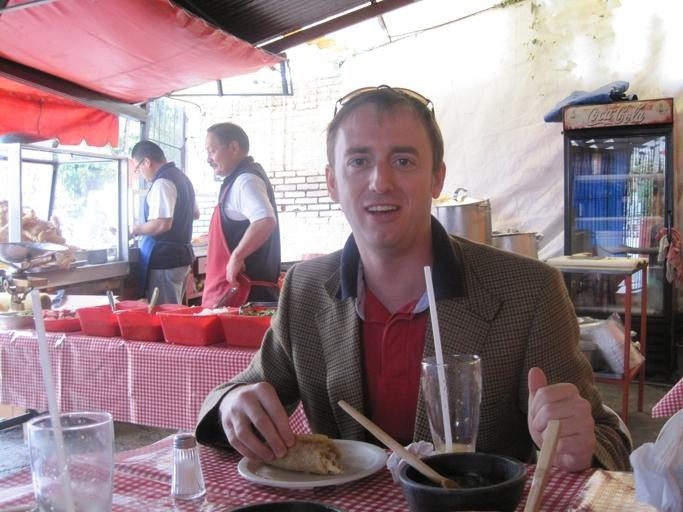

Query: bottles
170,433,206,501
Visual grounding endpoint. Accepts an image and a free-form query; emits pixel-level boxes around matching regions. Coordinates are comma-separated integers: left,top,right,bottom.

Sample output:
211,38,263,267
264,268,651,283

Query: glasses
134,159,144,174
334,84,434,116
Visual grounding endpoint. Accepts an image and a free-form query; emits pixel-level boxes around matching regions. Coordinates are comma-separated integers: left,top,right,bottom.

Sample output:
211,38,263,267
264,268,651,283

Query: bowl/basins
396,452,528,511
220,498,346,511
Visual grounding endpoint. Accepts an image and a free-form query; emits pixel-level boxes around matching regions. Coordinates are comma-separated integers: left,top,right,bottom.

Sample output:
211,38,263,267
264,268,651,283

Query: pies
262,432,344,476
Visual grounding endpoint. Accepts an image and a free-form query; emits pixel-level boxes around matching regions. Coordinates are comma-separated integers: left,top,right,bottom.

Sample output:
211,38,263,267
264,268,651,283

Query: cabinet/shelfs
552,255,649,422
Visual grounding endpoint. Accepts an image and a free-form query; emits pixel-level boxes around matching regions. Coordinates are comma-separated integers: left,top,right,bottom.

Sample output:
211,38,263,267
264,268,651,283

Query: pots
432,187,545,261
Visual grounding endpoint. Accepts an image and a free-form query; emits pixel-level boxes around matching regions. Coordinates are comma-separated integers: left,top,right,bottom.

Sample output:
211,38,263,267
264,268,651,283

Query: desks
1,329,312,439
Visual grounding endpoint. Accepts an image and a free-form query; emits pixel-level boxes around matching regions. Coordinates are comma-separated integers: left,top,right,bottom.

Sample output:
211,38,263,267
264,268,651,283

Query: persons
126,141,199,306
199,123,281,309
195,85,632,476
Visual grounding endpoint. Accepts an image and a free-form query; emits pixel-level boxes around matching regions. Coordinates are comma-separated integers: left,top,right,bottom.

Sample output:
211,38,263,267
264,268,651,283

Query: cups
24,410,116,511
417,354,485,456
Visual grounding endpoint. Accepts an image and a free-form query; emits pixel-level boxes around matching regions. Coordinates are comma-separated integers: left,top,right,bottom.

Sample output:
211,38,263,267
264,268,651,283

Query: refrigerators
562,98,676,385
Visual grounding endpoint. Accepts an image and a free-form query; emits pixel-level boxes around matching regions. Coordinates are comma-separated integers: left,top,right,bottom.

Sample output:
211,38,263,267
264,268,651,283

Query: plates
237,440,388,489
